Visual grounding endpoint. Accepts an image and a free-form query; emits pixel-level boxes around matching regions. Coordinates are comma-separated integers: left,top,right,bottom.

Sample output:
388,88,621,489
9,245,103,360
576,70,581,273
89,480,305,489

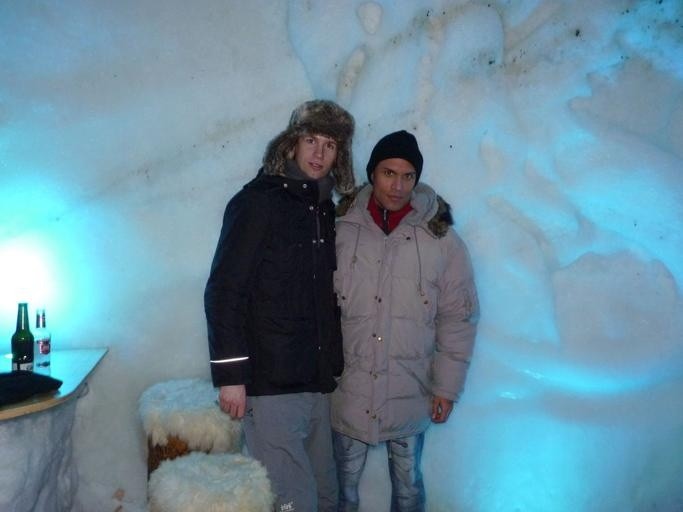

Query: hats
262,99,356,195
366,131,423,188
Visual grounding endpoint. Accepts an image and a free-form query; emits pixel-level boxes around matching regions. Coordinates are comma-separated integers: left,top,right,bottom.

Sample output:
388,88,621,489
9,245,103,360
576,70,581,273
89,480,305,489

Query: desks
0,344,109,511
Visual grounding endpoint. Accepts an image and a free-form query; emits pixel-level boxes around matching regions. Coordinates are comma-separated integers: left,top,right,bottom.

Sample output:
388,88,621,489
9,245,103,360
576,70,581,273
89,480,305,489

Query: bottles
32,304,51,374
11,303,34,373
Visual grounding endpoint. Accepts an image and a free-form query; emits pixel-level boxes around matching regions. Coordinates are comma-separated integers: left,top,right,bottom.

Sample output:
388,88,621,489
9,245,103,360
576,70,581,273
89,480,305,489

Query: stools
141,378,246,474
143,452,274,510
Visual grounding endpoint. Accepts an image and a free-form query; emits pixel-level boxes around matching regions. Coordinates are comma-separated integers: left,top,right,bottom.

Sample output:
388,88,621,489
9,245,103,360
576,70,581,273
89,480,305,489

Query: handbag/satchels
0,369,62,406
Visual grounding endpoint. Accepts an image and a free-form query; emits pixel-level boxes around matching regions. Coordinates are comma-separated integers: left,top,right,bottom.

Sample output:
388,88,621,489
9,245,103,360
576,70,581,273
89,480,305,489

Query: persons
329,125,477,512
200,97,355,511
